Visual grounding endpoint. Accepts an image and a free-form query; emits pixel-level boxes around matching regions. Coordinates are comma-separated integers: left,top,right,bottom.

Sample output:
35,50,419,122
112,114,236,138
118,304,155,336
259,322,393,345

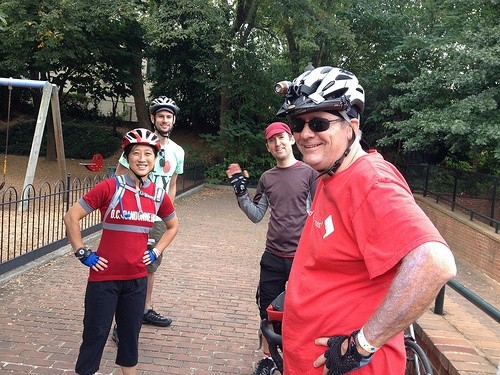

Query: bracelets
151,248,160,258
358,326,380,353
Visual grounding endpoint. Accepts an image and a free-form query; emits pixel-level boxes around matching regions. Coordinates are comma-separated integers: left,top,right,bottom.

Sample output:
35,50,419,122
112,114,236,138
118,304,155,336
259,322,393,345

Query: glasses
288,118,345,132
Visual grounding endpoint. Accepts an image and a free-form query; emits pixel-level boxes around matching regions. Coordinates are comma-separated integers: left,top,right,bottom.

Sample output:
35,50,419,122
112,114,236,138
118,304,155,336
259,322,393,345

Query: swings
0,85,12,190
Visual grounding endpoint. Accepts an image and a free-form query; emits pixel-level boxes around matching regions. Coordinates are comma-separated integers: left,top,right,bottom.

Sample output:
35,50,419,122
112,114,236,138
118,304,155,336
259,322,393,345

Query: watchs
74,246,88,258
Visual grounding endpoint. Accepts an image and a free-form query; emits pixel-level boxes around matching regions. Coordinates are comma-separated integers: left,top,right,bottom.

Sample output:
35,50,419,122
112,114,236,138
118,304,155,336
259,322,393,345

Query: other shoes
251,356,275,375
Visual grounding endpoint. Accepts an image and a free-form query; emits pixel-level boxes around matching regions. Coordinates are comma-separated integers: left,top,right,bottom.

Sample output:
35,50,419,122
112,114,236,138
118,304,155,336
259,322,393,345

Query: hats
263,122,291,140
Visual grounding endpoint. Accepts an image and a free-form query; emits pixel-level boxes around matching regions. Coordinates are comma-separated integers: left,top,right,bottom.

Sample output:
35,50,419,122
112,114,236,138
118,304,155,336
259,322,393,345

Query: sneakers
112,324,119,344
142,309,172,327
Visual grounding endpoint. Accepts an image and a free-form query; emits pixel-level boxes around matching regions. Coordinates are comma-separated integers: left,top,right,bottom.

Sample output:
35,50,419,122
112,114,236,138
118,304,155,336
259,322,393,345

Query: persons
63,127,179,375
116,97,185,345
275,66,458,375
226,123,321,375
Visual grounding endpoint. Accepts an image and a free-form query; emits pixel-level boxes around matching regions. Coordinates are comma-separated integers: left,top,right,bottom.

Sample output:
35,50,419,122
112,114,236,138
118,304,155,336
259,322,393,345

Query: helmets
121,128,161,152
275,66,366,118
149,96,180,115
